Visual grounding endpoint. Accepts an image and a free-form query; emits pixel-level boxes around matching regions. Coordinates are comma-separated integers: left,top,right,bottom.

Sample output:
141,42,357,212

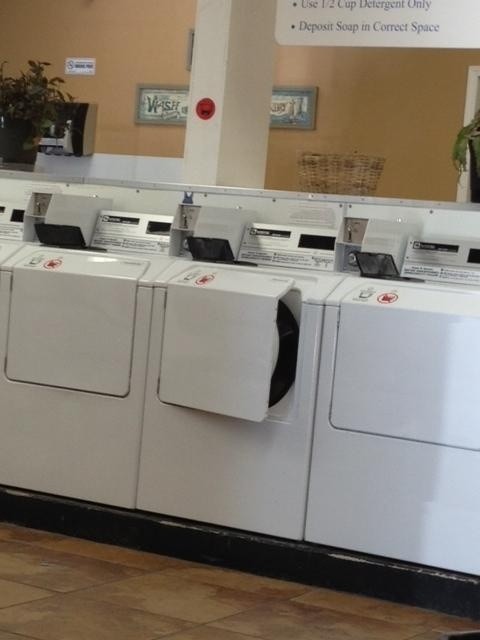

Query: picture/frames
131,80,320,131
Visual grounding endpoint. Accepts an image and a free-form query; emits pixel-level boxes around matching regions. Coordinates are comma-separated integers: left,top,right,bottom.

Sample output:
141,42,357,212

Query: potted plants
1,59,79,166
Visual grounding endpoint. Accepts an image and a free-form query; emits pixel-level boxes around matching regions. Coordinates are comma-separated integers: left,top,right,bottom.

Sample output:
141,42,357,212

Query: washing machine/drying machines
0,189,480,578
